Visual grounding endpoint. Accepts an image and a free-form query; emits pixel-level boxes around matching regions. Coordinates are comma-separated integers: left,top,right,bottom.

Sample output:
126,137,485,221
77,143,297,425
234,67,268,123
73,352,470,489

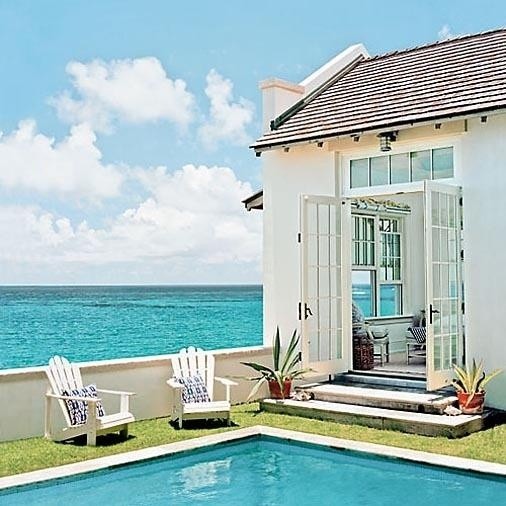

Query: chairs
165,347,239,429
43,355,136,447
352,299,390,368
406,310,462,367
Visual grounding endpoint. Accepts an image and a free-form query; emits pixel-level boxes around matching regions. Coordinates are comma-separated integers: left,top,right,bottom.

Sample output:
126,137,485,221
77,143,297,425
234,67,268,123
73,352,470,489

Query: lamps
377,132,398,151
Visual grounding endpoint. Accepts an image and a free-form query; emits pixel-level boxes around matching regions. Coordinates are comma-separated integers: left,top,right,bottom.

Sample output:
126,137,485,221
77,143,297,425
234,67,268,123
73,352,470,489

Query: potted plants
225,326,318,404
444,356,505,416
